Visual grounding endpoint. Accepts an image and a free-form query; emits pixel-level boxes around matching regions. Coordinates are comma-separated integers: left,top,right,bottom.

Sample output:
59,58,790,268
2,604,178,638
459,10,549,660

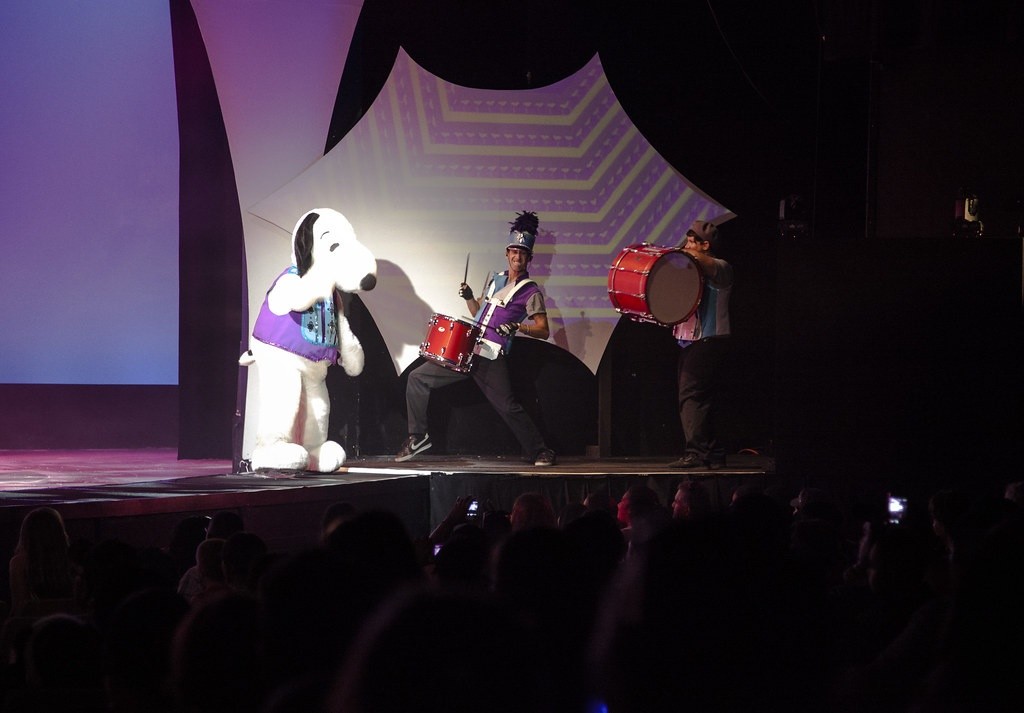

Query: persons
0,481,1024,713
395,245,558,465
667,221,735,470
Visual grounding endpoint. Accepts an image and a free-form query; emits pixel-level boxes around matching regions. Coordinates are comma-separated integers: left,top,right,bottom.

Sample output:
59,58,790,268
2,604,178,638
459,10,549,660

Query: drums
418,312,483,374
607,239,706,328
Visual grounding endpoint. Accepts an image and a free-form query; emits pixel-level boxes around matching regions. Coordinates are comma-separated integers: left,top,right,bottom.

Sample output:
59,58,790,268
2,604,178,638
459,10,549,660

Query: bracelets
522,322,530,335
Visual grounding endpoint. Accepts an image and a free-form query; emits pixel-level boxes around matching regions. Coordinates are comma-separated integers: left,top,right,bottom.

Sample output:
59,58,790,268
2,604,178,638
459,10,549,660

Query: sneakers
535,449,555,465
668,453,721,471
394,432,432,463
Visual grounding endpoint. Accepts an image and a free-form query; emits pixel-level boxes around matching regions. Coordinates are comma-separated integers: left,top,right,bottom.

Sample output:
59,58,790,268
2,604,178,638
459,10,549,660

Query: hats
505,212,539,251
690,220,719,245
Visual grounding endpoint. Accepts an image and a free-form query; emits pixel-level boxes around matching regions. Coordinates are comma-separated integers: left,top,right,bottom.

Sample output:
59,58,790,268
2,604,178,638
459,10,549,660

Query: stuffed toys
236,207,379,471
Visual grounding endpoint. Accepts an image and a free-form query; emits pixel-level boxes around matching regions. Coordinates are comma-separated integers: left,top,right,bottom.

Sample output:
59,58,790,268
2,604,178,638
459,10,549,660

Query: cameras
467,499,479,517
887,491,908,525
433,544,444,557
